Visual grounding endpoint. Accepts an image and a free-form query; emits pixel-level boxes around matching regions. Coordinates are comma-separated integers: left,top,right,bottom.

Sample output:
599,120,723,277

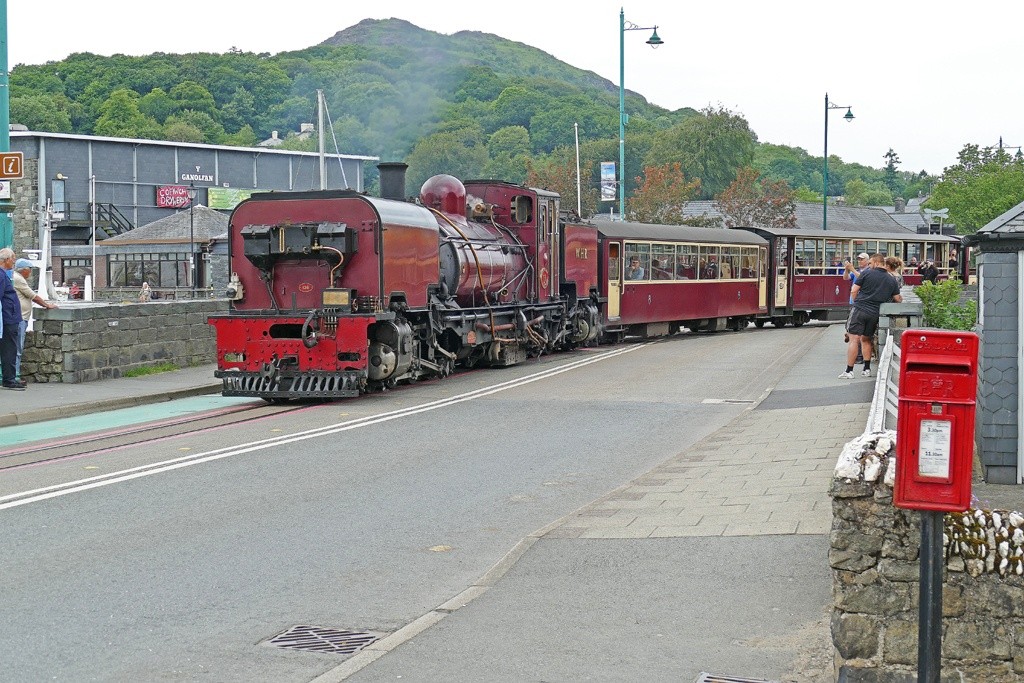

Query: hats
835,254,840,258
15,258,33,268
857,253,869,260
700,257,705,262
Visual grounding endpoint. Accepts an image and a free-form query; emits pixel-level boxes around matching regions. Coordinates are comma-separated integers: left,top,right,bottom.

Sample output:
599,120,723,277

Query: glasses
633,262,638,263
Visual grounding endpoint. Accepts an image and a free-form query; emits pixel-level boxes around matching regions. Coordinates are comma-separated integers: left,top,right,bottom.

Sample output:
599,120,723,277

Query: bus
203,175,964,401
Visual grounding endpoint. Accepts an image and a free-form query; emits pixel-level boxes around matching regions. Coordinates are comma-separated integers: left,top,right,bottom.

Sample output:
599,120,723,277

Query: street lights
823,104,857,225
186,182,201,289
618,25,665,219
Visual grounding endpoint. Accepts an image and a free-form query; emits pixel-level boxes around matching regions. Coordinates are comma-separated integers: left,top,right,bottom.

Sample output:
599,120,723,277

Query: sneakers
861,369,871,377
838,368,855,379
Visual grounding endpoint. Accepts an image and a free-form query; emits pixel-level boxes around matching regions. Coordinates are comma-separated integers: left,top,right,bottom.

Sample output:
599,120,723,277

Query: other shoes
871,353,877,361
1,380,26,391
17,378,27,387
855,356,863,363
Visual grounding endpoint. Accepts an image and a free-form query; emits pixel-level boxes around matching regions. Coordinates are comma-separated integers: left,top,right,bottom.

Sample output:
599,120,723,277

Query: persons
905,256,939,285
949,249,960,274
0,248,59,392
838,252,904,379
781,251,845,275
971,280,976,285
625,256,644,280
62,281,79,299
